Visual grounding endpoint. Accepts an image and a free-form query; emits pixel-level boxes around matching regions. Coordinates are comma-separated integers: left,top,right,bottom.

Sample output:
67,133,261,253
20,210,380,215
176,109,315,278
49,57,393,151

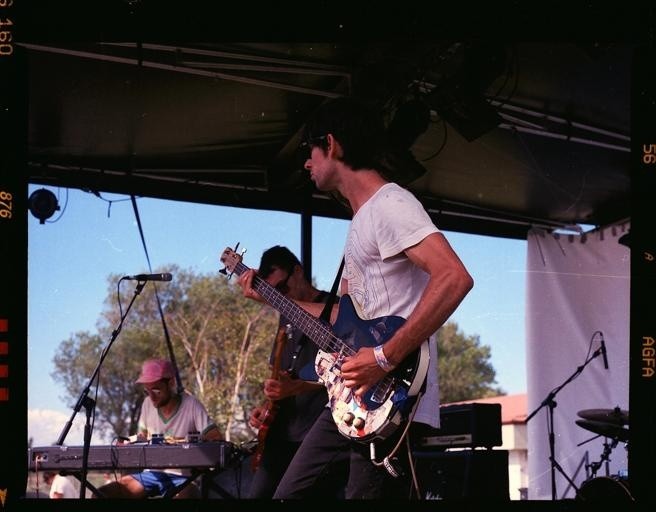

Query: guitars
252,326,286,465
218,243,430,448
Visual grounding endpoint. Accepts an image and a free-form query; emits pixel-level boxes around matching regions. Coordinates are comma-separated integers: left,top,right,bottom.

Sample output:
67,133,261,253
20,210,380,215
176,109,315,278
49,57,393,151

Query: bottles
152,434,164,445
187,431,201,444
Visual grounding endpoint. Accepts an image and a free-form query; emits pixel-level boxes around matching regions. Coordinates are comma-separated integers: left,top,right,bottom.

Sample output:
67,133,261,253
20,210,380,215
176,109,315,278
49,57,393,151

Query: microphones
600,332,608,369
122,273,172,281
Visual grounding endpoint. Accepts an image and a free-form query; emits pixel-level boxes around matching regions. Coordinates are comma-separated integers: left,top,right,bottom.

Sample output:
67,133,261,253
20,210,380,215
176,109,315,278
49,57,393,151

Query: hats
135,360,171,384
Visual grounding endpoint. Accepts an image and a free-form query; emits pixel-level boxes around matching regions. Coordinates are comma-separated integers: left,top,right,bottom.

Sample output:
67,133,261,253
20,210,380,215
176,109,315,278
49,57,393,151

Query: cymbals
577,408,630,425
575,419,629,444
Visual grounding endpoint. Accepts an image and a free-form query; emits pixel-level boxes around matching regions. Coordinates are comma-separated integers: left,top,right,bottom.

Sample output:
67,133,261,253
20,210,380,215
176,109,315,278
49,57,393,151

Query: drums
574,476,636,504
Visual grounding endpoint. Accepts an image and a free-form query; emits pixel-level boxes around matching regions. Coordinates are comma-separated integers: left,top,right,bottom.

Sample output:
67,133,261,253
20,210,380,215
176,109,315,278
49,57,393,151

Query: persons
250,246,341,500
43,470,79,499
91,358,225,499
238,117,474,500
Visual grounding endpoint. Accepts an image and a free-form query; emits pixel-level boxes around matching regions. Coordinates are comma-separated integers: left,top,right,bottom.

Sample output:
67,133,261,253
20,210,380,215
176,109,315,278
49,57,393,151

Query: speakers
407,451,509,501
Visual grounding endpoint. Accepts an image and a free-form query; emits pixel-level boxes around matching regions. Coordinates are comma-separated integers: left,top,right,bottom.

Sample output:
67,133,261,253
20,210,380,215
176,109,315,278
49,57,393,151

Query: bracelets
373,346,395,373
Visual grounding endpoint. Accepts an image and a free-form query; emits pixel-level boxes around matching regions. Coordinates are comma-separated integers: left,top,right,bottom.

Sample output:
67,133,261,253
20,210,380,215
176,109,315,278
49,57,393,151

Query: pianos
30,442,232,471
